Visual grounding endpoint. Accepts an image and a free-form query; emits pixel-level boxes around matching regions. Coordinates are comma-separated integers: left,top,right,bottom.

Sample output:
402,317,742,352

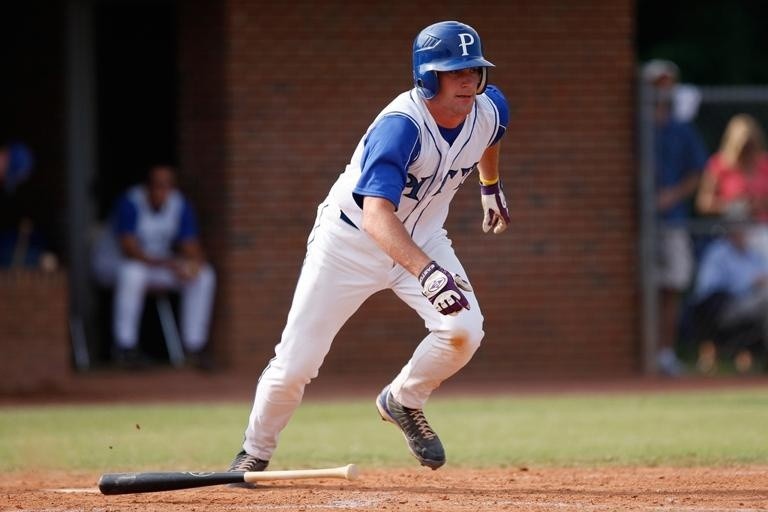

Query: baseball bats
98,463,361,494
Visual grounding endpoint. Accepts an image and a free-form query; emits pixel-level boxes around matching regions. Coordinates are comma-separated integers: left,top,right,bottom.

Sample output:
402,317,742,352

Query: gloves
418,261,472,316
479,176,512,234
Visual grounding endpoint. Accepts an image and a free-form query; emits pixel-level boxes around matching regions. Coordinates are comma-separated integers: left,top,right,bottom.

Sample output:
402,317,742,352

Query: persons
89,155,219,376
645,57,767,375
0,140,60,276
226,20,512,488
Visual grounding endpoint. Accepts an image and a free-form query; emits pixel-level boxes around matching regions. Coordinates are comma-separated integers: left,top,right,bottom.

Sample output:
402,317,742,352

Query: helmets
413,20,495,100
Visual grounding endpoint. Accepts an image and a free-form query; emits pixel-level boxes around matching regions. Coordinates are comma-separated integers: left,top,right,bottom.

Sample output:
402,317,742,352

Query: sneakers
376,385,445,470
225,450,269,487
